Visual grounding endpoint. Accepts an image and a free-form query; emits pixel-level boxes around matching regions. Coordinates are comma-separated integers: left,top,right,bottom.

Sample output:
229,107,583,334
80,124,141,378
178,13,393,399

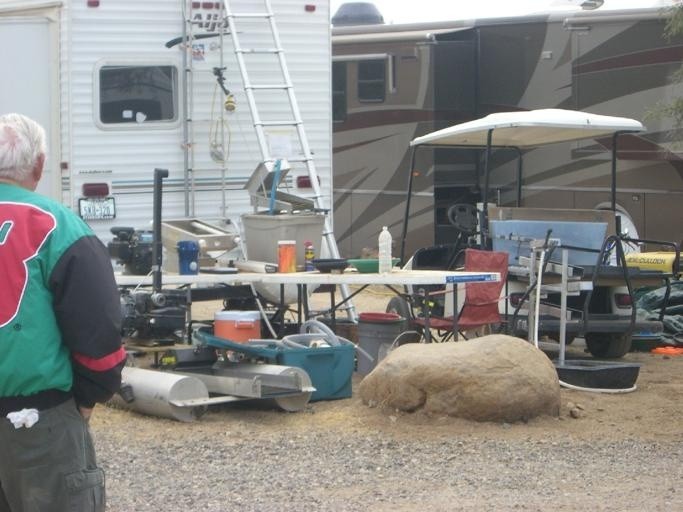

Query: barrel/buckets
357,311,400,375
214,310,262,344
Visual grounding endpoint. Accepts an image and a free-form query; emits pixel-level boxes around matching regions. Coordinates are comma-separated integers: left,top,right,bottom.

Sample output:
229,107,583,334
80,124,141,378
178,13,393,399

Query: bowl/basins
347,259,400,273
312,259,350,274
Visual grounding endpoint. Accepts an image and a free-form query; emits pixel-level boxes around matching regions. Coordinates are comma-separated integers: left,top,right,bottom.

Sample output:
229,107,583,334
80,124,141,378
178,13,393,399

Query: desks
112,270,500,367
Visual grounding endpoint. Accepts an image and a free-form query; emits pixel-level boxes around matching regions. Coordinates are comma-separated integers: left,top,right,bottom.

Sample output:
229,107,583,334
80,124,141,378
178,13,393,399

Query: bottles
378,226,392,273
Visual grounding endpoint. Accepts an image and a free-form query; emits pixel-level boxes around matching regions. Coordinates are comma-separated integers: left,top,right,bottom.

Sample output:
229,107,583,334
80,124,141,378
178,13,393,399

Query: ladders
224,1,356,323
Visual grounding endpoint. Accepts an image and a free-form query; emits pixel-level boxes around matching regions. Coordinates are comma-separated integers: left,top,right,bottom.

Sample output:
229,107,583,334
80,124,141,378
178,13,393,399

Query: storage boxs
240,212,326,271
192,310,355,402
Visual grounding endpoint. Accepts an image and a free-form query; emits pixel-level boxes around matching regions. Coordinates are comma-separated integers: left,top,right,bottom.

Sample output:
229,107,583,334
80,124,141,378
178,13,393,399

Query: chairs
410,248,509,348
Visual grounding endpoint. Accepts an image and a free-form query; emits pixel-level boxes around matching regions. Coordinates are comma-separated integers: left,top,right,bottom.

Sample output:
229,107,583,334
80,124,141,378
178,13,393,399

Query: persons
1,113,126,512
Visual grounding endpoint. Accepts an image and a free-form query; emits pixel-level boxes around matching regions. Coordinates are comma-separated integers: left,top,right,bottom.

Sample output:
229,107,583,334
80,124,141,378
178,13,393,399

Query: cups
175,241,199,274
277,240,296,274
